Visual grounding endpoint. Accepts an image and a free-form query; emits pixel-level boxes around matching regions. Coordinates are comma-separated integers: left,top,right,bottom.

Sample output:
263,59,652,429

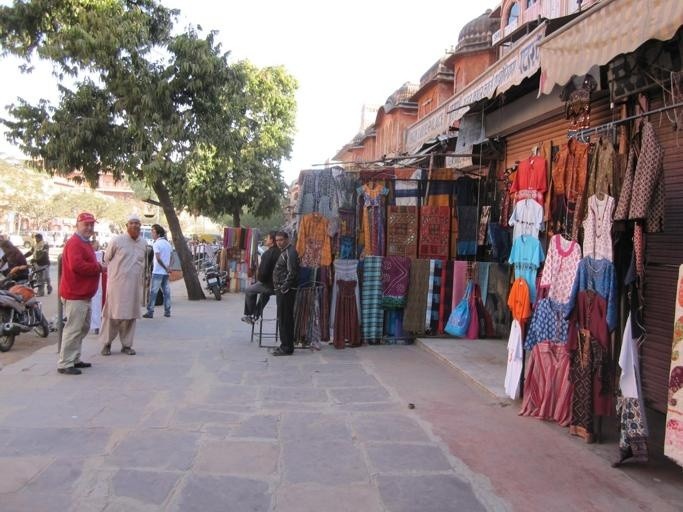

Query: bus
123,225,167,250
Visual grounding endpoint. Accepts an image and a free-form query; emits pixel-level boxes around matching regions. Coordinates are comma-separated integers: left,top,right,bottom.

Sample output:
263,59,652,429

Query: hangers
556,230,575,243
586,245,606,263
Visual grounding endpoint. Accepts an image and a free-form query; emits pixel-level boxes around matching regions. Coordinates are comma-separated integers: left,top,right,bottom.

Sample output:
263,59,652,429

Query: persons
270,231,300,355
0,233,29,291
141,226,174,318
54,212,107,374
146,222,168,307
97,212,151,355
240,230,280,325
186,237,222,262
23,233,52,297
89,231,105,263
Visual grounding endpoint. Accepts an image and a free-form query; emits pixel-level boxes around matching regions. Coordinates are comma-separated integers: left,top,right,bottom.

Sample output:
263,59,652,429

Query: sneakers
241,314,260,324
74,361,92,368
119,344,134,355
47,285,52,296
142,312,154,318
99,343,112,357
58,367,81,375
270,344,296,357
163,311,170,316
34,292,44,297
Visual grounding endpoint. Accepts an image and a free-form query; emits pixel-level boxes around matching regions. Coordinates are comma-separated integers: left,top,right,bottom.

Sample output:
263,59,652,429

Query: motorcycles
192,249,230,300
0,263,51,351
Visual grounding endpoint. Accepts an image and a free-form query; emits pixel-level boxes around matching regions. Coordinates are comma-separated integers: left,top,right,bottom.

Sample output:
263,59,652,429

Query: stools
249,290,280,348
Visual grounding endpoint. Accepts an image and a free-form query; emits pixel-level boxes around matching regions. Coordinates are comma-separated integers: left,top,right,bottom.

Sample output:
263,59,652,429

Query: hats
76,210,98,224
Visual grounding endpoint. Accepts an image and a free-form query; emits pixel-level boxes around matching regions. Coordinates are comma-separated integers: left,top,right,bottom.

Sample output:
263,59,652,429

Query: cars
0,215,117,249
